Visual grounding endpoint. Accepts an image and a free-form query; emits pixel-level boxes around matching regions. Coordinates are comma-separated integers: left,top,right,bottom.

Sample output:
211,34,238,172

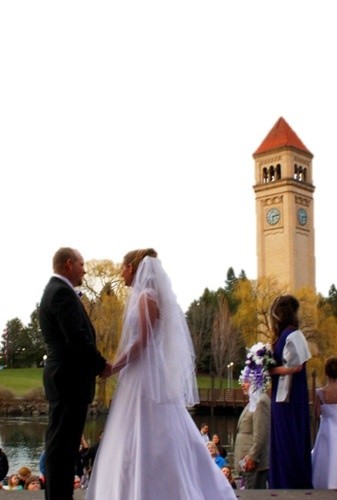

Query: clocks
263,203,284,231
296,203,311,232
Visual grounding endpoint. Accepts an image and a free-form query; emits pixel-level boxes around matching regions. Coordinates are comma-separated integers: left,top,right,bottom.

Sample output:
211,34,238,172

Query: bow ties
78,292,83,296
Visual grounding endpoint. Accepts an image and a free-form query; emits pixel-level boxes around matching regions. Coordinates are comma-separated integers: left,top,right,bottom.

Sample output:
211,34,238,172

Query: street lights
230,362,233,388
227,365,230,388
43,355,48,366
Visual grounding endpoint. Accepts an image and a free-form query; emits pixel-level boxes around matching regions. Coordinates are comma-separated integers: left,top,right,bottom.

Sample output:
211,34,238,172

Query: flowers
238,342,275,395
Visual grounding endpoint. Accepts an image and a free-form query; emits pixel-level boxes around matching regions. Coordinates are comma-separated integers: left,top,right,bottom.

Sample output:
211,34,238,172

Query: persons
84,247,238,499
4,474,23,490
73,473,80,490
205,441,228,466
37,246,111,500
267,294,314,489
199,423,209,439
23,475,42,491
221,466,237,489
310,357,337,489
211,433,228,457
232,380,272,489
17,466,31,482
